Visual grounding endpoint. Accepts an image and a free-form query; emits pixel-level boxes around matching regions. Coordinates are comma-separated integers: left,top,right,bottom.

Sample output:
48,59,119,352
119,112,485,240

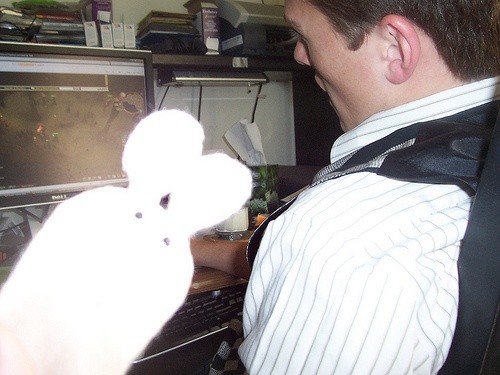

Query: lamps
157,70,269,86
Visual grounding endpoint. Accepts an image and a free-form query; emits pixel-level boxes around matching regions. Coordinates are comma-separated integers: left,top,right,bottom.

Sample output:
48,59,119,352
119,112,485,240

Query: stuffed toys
0,110,252,375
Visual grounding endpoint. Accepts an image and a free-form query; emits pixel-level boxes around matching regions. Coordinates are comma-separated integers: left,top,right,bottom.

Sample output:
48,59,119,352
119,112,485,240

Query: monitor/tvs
0,41,156,212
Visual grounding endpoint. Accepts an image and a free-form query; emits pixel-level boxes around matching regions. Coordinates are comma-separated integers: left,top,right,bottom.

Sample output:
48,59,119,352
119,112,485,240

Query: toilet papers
215,207,249,233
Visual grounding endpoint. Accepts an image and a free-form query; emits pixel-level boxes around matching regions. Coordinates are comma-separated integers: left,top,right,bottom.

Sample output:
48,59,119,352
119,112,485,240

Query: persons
190,0,500,375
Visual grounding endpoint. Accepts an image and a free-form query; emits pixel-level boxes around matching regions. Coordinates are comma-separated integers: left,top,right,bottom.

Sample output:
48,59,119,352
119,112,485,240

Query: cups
249,164,280,227
216,201,249,239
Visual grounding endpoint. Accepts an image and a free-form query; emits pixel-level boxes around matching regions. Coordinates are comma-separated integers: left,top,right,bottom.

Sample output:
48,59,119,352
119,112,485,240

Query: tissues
223,118,280,202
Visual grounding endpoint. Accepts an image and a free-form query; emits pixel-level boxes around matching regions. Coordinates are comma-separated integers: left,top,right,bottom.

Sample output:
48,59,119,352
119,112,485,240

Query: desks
188,229,255,295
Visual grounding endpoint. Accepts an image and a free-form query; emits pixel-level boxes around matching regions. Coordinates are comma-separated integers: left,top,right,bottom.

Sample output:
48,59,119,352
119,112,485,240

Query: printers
218,0,297,57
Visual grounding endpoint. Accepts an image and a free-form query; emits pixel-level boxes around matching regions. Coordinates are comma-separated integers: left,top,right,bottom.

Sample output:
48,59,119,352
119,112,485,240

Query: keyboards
133,282,249,364
